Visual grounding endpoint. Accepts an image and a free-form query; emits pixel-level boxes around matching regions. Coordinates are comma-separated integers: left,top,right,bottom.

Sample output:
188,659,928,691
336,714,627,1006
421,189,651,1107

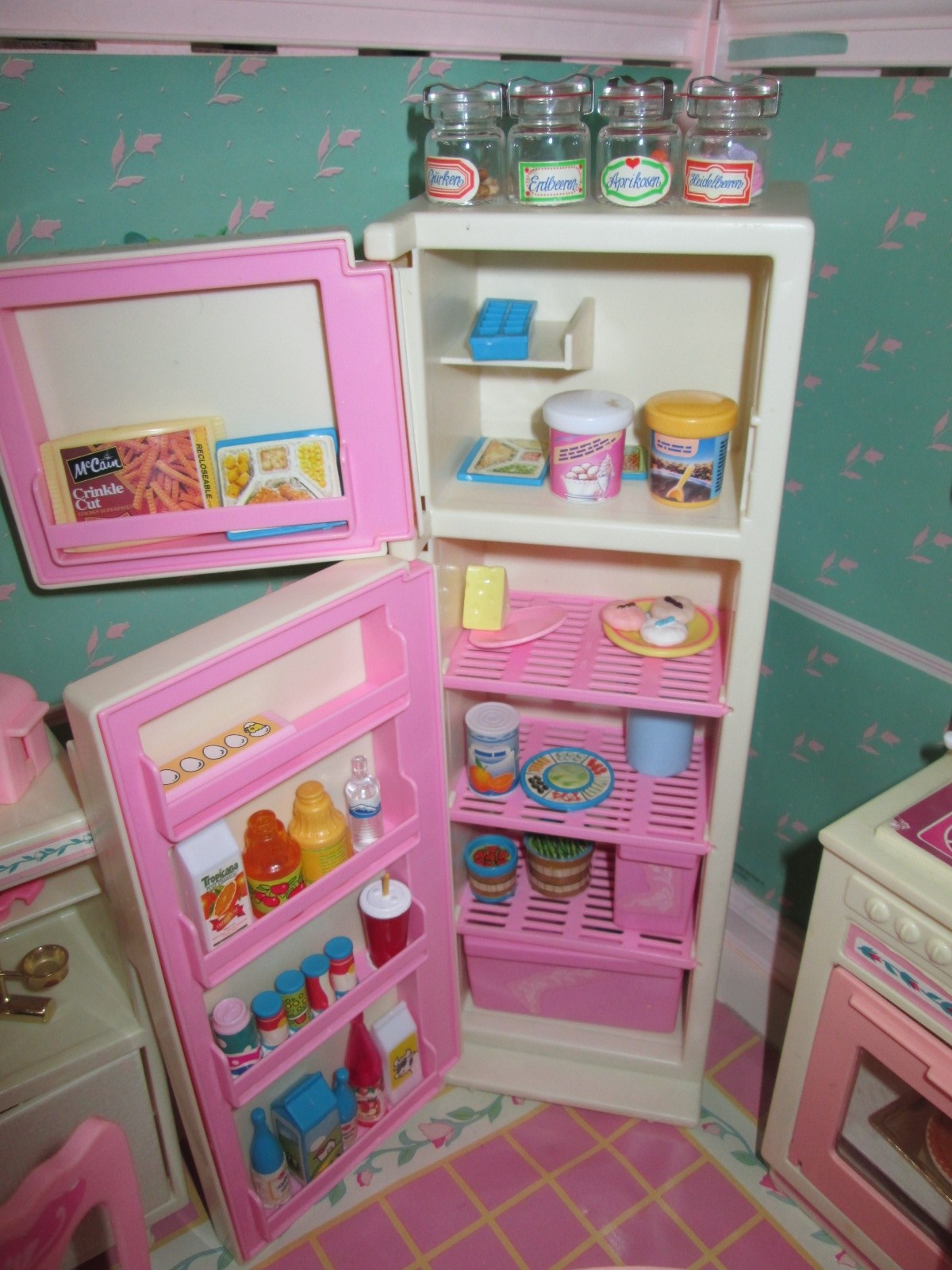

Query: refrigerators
0,175,812,1264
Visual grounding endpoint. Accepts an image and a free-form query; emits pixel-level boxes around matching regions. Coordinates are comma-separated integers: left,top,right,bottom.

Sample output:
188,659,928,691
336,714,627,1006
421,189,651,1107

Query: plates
468,604,571,652
604,596,717,658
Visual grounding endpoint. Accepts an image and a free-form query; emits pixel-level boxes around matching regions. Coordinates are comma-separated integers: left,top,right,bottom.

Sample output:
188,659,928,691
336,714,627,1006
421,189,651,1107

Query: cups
359,879,413,970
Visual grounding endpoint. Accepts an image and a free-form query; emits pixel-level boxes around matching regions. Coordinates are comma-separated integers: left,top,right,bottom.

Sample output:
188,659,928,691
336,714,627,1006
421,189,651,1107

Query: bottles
347,1010,391,1129
253,1104,293,1216
331,1067,359,1147
347,756,387,855
294,781,348,887
241,808,304,919
417,76,781,211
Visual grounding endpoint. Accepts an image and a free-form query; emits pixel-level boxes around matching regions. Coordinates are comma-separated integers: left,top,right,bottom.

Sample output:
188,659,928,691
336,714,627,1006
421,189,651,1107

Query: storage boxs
611,842,706,937
463,934,687,1038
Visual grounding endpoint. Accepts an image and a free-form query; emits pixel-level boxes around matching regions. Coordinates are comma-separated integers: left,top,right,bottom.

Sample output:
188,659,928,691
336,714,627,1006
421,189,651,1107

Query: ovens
760,754,952,1270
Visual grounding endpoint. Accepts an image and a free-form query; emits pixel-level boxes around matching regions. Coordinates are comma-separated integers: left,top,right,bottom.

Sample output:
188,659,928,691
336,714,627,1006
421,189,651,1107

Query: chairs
0,1113,153,1270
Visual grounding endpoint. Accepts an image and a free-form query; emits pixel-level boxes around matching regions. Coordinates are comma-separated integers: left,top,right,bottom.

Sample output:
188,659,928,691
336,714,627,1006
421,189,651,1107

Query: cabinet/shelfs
0,714,189,1270
